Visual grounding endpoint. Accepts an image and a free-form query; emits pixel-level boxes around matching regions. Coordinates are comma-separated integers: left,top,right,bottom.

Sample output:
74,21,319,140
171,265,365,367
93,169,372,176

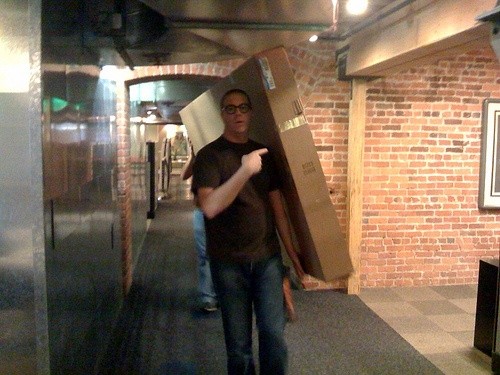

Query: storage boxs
178,46,355,282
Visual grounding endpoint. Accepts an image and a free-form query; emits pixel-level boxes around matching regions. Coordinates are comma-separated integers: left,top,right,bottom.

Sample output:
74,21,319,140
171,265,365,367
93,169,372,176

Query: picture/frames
478,98,500,210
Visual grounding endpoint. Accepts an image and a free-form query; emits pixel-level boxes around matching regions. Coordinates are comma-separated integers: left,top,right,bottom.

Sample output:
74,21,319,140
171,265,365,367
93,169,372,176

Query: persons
182,89,305,375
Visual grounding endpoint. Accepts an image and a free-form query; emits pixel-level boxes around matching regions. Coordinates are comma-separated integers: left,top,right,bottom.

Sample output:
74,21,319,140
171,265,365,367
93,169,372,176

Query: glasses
224,104,250,115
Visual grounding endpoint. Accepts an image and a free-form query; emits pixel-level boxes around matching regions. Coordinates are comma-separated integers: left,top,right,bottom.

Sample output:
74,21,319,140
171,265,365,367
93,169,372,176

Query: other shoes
189,304,216,318
